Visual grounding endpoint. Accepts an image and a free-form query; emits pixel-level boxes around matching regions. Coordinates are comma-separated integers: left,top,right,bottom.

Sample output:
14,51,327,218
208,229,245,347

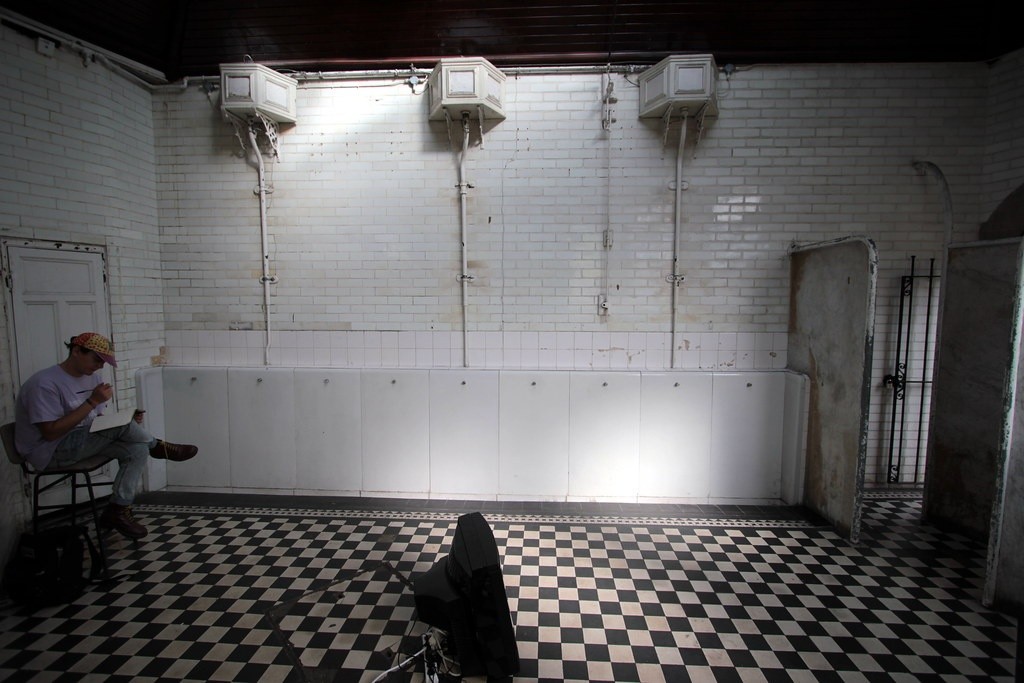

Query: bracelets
86,399,96,409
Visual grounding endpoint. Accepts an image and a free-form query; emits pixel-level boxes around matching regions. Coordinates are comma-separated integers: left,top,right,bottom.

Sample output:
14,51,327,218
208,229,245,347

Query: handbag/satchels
3,523,105,603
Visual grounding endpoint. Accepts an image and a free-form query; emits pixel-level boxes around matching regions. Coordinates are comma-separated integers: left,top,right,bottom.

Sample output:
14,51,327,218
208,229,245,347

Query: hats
73,332,117,369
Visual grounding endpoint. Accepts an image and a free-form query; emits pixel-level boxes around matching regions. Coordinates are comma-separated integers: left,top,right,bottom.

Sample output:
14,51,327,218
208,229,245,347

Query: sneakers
149,438,198,462
99,504,148,538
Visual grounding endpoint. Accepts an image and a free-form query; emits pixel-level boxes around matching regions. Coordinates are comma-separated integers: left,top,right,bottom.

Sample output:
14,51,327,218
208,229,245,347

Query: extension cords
422,634,439,683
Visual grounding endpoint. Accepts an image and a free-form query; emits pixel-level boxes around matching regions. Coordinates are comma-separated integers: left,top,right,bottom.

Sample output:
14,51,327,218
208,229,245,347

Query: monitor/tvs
414,512,520,677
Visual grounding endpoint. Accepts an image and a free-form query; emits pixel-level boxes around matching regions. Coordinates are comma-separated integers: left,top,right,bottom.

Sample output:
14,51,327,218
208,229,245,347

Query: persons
15,332,198,540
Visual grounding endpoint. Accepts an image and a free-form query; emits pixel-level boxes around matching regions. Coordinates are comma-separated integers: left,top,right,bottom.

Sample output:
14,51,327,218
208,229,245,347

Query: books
90,408,145,432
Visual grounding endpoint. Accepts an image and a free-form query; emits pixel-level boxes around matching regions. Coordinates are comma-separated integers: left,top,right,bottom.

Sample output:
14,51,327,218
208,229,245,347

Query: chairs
1,422,138,575
